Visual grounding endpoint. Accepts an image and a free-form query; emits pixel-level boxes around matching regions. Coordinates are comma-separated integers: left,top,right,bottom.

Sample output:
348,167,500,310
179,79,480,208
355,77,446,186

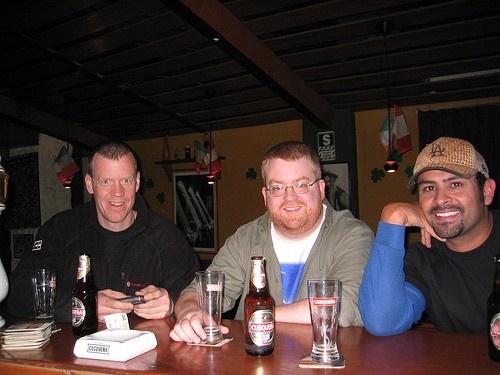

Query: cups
305,278,341,365
195,270,224,345
31,267,57,329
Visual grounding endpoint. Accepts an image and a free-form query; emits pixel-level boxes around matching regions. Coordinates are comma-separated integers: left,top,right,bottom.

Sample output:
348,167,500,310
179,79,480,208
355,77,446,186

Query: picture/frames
172,169,219,255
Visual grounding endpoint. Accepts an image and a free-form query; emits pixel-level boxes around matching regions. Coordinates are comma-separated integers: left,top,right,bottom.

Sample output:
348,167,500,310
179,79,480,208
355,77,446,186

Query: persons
5,140,201,323
359,136,499,337
170,141,376,344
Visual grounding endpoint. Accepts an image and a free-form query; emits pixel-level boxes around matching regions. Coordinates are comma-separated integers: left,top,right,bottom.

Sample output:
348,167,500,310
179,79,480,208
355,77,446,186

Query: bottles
486,254,500,363
243,256,275,356
71,253,99,337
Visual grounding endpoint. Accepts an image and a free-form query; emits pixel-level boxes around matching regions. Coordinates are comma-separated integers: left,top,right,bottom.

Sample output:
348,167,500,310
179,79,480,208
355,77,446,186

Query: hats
406,137,489,194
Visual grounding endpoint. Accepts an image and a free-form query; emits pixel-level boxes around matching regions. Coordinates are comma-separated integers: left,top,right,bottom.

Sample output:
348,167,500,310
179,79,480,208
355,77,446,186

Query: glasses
267,178,320,197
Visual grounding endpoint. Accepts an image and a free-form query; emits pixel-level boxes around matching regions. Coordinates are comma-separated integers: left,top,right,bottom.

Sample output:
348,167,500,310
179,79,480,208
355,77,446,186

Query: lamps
383,107,399,173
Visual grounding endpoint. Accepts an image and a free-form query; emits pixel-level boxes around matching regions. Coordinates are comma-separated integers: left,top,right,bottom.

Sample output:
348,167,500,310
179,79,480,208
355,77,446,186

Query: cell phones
115,295,146,305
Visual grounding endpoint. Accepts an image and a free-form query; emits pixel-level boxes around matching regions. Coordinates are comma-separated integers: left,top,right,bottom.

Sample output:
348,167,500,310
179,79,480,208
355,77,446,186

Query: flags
54,147,79,181
194,139,223,174
378,105,412,164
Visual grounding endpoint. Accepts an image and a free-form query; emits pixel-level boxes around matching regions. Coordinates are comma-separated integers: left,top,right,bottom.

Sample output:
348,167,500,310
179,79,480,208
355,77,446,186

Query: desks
0,317,500,375
